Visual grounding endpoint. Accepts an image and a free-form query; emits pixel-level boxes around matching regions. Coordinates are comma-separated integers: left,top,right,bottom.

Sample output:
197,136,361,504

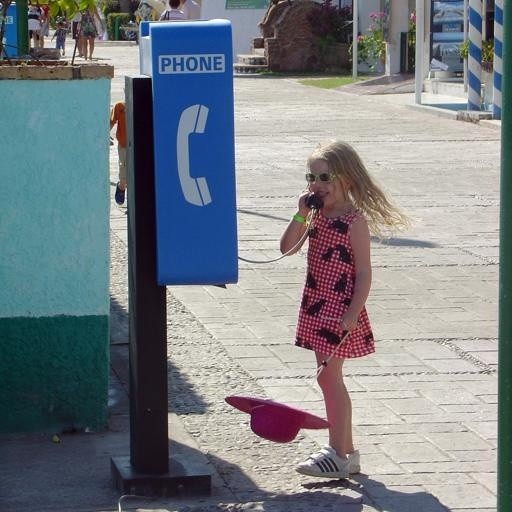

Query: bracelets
292,214,307,224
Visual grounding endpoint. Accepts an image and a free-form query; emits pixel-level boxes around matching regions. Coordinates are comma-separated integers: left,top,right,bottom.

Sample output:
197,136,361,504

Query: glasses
305,173,337,182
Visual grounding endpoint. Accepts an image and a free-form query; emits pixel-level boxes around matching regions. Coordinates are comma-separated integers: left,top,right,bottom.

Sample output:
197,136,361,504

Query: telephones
305,192,323,209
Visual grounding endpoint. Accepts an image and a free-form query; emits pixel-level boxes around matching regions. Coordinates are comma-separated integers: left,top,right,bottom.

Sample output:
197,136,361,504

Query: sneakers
295,445,361,478
115,182,125,205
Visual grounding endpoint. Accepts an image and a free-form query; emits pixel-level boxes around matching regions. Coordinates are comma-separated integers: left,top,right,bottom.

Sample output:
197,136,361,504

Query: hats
225,397,328,443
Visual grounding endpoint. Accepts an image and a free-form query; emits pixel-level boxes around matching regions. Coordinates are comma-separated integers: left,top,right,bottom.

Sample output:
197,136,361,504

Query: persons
110,100,128,205
28,2,105,61
159,0,201,21
280,139,415,479
